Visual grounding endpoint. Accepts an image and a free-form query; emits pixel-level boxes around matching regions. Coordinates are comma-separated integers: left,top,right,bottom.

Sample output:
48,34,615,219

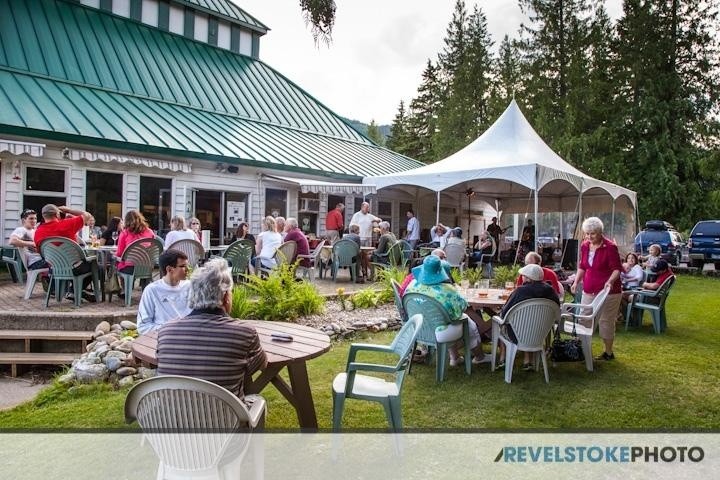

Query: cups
474,279,489,299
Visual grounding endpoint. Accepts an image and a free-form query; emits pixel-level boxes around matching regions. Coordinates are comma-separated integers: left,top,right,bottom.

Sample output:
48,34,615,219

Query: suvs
634,221,687,267
688,219,720,270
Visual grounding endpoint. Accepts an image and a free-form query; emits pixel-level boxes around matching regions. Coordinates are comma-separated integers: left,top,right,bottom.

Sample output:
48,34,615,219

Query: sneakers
472,353,492,364
43,274,133,304
450,356,464,366
495,346,551,371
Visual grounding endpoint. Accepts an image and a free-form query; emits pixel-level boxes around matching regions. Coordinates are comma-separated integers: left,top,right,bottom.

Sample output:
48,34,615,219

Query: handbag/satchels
103,270,120,294
551,339,585,362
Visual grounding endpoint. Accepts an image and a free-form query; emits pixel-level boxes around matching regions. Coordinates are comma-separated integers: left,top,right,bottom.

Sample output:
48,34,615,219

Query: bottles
91,228,101,249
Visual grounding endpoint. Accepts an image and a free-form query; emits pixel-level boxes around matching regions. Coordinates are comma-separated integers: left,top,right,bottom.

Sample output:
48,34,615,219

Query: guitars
493,225,513,236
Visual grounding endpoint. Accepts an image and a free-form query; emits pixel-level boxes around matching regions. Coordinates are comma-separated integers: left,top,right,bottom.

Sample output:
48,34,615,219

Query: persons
137,248,204,329
403,254,492,367
4,200,666,305
570,216,623,362
154,255,269,466
626,260,675,328
494,263,560,373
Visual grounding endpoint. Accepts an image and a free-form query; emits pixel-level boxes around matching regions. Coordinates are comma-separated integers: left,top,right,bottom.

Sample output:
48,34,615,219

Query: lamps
215,163,226,172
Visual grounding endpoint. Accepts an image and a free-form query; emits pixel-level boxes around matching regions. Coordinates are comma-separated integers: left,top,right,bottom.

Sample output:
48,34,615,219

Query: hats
518,264,544,281
42,204,63,213
411,255,451,284
651,260,668,273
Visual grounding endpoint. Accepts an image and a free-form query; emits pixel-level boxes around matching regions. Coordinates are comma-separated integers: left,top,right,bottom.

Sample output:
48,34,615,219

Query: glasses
24,210,35,215
176,263,190,267
192,223,199,225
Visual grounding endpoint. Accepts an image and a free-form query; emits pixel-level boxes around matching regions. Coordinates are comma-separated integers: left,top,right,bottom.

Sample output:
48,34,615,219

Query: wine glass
505,281,515,296
112,231,119,247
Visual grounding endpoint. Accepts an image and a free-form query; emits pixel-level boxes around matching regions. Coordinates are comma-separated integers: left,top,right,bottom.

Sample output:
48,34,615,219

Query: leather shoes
595,352,616,361
